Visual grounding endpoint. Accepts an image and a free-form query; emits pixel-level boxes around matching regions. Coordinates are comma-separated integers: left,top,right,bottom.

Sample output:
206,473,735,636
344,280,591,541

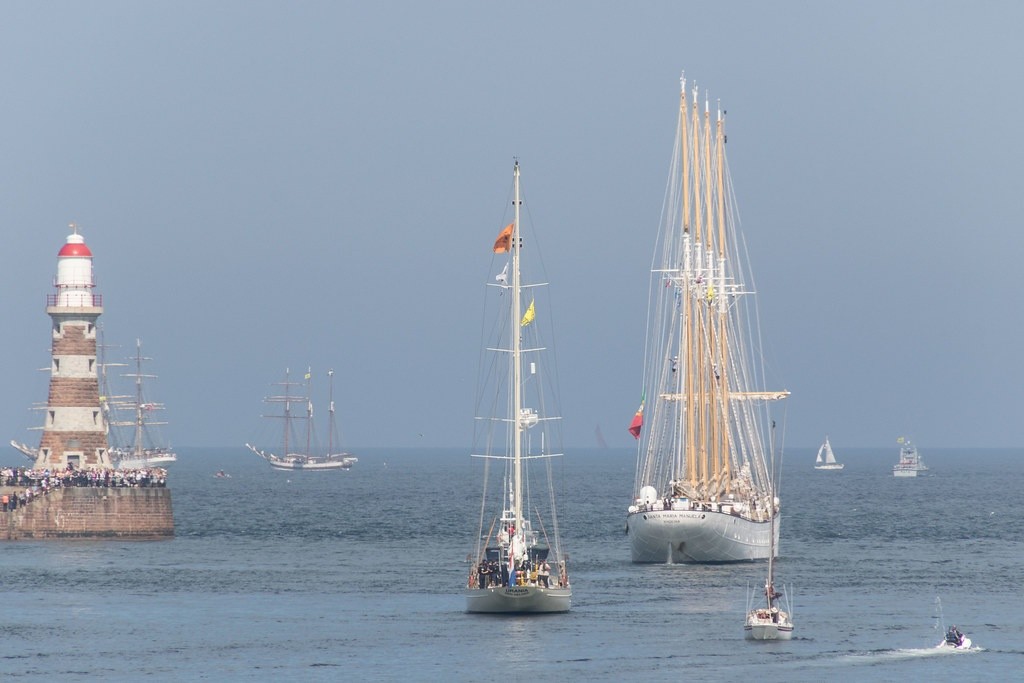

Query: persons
467,559,568,590
949,626,959,639
763,584,777,608
0,448,176,513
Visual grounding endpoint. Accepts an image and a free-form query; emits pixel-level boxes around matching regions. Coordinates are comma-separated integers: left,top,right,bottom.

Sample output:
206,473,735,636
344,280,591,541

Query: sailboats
242,362,358,471
624,68,792,567
812,433,845,470
465,153,572,615
893,437,929,477
742,420,800,641
10,322,180,472
933,596,972,653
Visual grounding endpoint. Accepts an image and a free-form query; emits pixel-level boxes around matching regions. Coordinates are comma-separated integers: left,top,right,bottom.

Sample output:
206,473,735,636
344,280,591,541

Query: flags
521,300,534,327
492,223,515,254
509,545,515,590
628,385,646,441
496,263,508,286
897,437,905,443
304,374,310,379
